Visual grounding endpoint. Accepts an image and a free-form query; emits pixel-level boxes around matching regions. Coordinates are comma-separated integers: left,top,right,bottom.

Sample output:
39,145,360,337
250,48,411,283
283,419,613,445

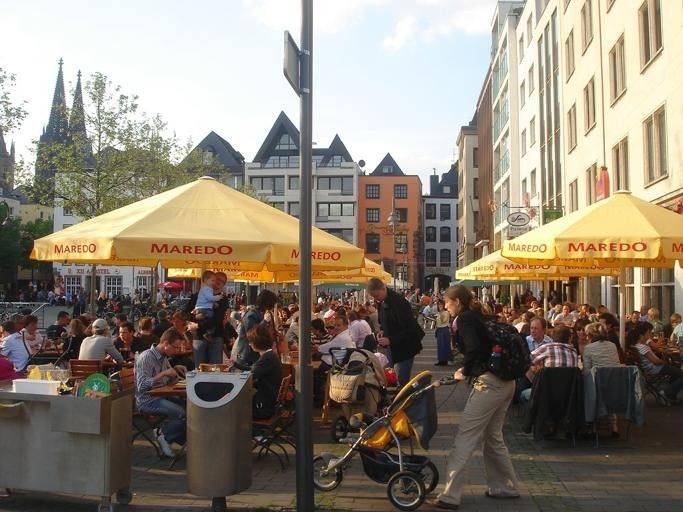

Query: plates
178,379,187,383
80,372,110,398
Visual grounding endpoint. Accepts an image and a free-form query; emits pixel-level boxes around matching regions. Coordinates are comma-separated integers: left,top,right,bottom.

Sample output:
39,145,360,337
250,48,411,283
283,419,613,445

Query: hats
92,318,110,331
320,291,326,299
231,311,240,321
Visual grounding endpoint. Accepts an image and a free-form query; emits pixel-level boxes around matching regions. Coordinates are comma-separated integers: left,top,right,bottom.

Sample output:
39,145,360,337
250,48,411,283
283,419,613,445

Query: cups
280,352,291,364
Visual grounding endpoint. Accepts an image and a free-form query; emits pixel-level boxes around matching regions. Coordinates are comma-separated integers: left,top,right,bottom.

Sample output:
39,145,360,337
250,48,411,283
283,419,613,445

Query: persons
309,318,333,351
527,300,542,317
670,322,683,343
434,300,455,367
646,322,666,351
229,289,277,368
280,295,348,323
549,290,561,305
371,352,397,386
624,313,631,320
625,312,642,346
367,277,425,391
409,288,422,320
510,310,521,323
1,321,35,370
19,310,33,323
519,312,536,337
114,325,149,358
582,322,622,369
629,325,683,407
21,317,46,346
554,303,581,324
284,312,301,348
325,319,337,337
317,317,356,400
137,319,156,338
525,318,552,352
186,270,229,372
529,326,578,368
547,301,555,318
1,282,183,316
111,314,135,335
539,290,545,304
193,270,224,345
225,325,282,422
598,313,620,351
663,313,681,338
424,285,522,509
77,319,127,364
587,306,599,322
422,302,436,330
639,306,649,320
482,303,492,318
582,304,589,320
62,319,90,360
46,311,68,339
346,310,375,350
597,305,609,314
132,327,186,456
554,303,562,318
171,313,192,341
644,308,665,331
156,311,173,336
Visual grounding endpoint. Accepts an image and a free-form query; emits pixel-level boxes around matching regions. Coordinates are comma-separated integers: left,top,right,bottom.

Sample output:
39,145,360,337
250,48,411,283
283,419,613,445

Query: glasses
576,327,586,332
118,329,130,335
170,344,181,350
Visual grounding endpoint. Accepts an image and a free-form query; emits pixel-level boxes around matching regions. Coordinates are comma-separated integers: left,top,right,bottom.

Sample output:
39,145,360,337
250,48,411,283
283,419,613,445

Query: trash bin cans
184,371,253,499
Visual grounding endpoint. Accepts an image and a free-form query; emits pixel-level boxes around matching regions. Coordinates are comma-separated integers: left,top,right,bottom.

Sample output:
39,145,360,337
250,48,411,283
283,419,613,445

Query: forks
176,373,184,380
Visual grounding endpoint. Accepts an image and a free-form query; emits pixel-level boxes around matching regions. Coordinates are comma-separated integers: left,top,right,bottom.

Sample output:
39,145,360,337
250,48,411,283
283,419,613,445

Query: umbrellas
456,217,624,326
26,176,364,286
452,278,527,288
251,271,394,298
500,190,683,356
165,252,388,297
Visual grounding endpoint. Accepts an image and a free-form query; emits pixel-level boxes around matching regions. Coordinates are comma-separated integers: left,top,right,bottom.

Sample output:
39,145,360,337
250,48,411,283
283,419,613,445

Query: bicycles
128,299,152,320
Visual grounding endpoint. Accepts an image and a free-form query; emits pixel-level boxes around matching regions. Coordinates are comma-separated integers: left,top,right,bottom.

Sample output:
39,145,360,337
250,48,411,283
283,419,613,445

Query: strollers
314,369,460,512
328,347,400,442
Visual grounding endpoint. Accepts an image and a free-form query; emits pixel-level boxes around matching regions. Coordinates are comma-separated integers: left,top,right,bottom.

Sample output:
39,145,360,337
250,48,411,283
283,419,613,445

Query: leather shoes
578,432,594,444
611,430,621,444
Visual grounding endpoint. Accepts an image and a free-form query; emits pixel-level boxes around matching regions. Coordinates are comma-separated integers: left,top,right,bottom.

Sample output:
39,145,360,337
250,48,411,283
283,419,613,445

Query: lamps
387,195,400,292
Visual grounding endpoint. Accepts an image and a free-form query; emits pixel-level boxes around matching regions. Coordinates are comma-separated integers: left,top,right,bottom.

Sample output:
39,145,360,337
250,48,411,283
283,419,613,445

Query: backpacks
471,307,530,381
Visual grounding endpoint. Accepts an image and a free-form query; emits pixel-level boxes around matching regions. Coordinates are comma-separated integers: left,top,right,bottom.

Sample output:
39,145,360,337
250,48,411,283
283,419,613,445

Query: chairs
587,366,642,450
69,359,103,378
627,347,668,407
254,373,293,469
110,367,166,462
534,366,583,450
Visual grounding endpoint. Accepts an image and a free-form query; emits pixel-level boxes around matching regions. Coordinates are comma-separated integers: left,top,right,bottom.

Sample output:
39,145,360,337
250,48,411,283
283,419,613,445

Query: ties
161,358,165,371
417,294,419,303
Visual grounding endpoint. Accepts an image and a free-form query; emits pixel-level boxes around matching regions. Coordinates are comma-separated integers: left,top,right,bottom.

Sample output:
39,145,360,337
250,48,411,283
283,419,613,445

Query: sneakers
156,434,176,459
152,427,159,441
423,497,459,511
657,390,671,406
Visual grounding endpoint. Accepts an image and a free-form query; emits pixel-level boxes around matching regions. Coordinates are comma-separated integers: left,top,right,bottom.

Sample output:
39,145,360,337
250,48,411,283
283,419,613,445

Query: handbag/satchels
328,373,360,405
